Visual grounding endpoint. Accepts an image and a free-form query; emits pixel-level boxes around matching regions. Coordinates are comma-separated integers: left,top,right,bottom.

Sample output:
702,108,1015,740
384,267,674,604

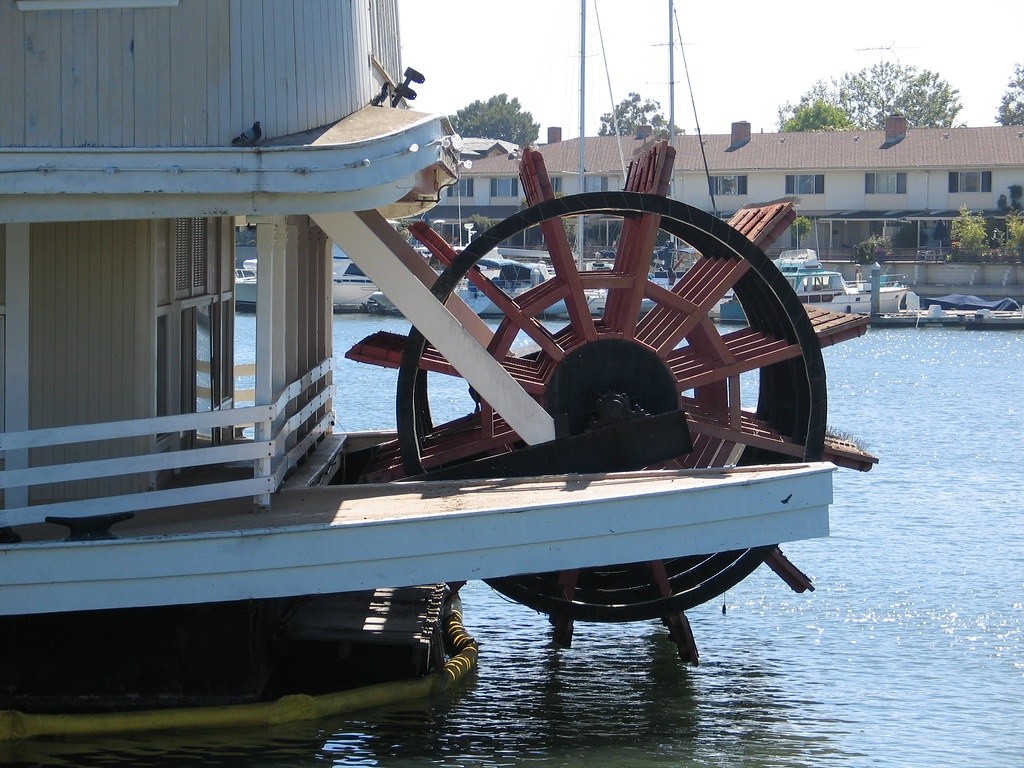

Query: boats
235,0,1023,337
